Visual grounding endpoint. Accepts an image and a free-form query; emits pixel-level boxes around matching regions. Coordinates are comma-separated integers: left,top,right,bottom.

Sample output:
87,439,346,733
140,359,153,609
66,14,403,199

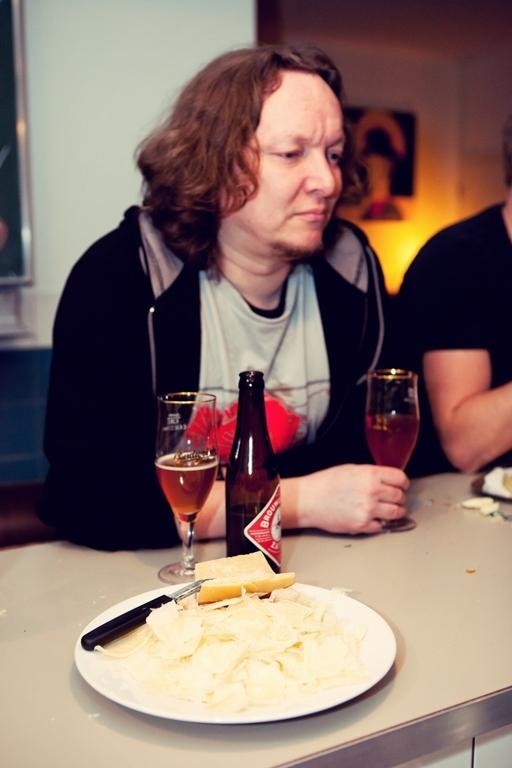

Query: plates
74,579,401,726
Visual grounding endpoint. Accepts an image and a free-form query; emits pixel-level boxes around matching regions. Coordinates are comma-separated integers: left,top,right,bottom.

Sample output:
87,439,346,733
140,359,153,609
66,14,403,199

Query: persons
388,123,511,481
35,40,427,542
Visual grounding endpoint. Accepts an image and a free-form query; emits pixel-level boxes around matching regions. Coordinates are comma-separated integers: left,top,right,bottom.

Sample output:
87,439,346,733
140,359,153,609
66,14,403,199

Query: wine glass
155,391,219,585
362,369,420,535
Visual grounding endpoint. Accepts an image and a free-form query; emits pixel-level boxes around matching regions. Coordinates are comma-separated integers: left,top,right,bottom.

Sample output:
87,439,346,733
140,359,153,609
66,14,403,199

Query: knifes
81,575,213,652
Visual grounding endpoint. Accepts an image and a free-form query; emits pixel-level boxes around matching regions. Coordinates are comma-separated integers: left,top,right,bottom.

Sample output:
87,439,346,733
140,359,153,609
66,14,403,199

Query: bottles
225,370,282,577
